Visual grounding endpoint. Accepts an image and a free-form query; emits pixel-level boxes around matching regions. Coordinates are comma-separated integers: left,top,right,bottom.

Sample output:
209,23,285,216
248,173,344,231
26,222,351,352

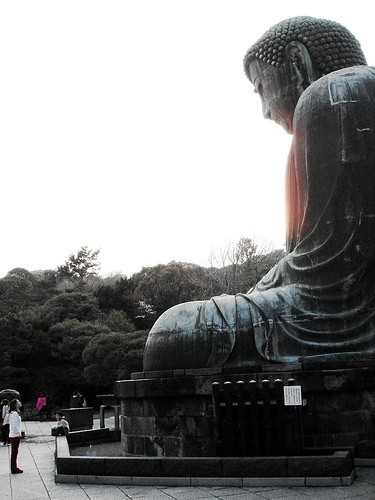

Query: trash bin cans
60,408,93,432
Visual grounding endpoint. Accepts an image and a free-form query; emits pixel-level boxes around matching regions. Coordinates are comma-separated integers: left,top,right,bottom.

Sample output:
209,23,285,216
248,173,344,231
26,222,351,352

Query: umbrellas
0,388,20,396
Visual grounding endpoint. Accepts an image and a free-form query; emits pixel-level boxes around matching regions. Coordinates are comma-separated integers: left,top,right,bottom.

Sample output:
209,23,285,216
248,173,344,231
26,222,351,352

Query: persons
0,399,23,474
51,412,69,438
37,396,47,422
70,392,85,407
143,16,375,372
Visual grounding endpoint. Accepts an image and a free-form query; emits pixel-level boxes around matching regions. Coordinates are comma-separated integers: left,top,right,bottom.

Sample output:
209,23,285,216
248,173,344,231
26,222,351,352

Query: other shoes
7,444,10,446
0,442,6,447
11,468,24,474
